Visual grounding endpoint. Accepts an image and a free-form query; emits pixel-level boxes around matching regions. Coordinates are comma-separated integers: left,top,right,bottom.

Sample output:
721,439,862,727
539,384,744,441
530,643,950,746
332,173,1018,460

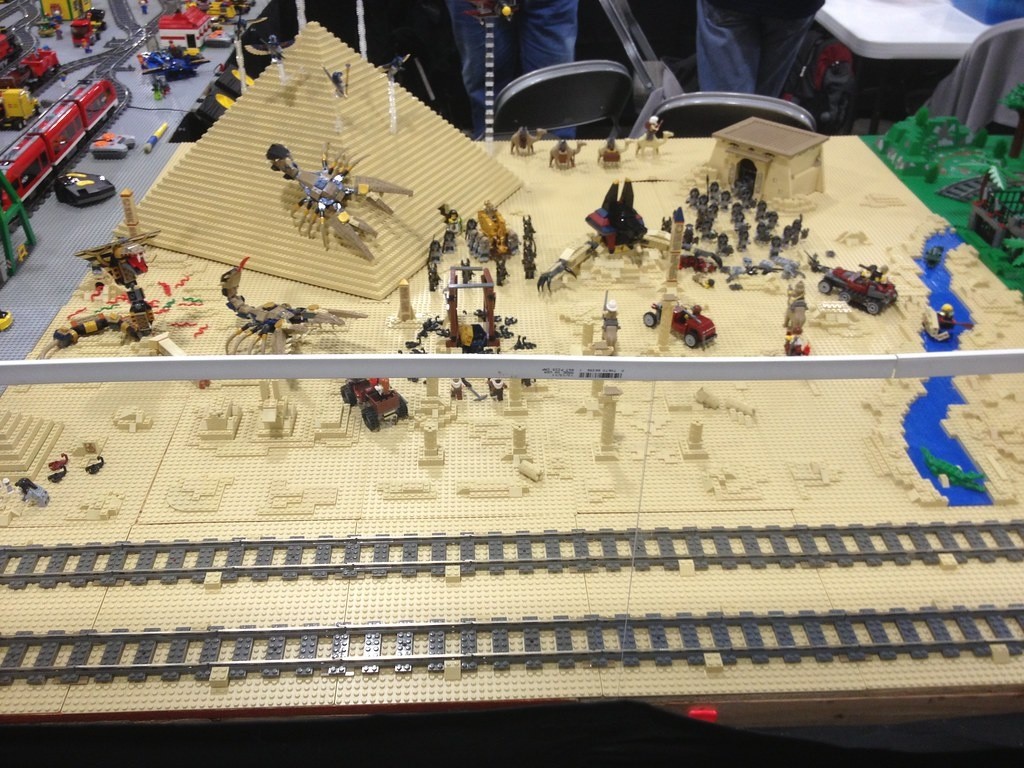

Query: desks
0,0,1024,735
815,1,992,138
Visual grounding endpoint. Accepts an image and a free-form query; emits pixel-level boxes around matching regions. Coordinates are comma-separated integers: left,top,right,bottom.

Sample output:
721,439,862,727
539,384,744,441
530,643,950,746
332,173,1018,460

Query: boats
938,299,954,342
923,244,944,270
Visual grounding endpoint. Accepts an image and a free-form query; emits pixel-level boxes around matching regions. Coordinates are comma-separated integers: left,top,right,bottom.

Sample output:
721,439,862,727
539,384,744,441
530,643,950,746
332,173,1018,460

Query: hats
941,304,954,312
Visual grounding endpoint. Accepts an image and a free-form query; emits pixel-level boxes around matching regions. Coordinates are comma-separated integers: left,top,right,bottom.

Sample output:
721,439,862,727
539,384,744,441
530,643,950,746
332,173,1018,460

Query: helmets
448,209,458,218
444,231,455,241
429,240,441,250
690,304,702,313
467,219,478,229
661,174,803,247
607,300,618,312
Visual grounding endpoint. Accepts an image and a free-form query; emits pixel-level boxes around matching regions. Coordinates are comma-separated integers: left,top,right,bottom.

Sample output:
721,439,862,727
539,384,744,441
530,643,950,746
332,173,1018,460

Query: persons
696,1,824,98
446,0,580,140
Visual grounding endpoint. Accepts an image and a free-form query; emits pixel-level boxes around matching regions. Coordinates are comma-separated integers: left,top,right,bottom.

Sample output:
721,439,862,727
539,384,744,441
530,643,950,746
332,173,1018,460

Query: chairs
651,90,818,139
599,1,686,139
953,16,1024,141
473,60,633,141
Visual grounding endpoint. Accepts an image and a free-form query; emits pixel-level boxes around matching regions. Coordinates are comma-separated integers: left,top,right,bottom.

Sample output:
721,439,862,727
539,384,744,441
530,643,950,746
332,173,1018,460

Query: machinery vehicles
679,250,723,272
643,300,717,350
0,0,245,207
818,263,895,315
339,376,410,429
